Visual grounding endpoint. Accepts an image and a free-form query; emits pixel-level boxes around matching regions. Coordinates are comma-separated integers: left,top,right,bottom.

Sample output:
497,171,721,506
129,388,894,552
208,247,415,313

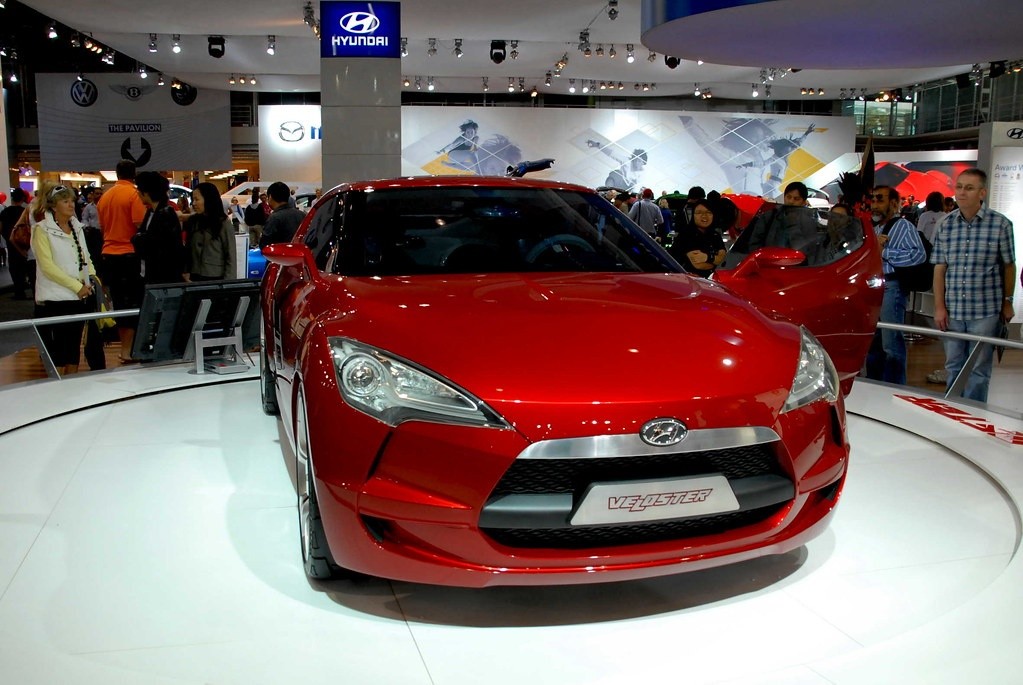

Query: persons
671,199,727,278
749,180,863,265
866,184,926,384
73,159,322,364
627,188,664,238
441,134,522,175
435,119,481,174
0,187,44,301
585,139,648,192
30,180,95,378
898,191,955,241
679,116,782,198
735,123,816,197
658,186,721,246
603,189,642,215
928,168,1016,404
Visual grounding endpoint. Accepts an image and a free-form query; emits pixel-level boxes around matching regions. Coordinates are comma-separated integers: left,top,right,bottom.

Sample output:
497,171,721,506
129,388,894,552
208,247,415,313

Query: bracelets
704,254,715,263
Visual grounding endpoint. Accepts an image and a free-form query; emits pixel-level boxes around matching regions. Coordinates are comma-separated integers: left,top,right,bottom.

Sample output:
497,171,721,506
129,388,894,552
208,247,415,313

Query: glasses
51,185,68,197
869,193,889,200
692,210,713,216
955,184,984,191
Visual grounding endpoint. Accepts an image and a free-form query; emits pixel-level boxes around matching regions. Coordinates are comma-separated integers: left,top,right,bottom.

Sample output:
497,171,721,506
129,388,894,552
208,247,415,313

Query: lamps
0,0,321,90
401,1,1021,102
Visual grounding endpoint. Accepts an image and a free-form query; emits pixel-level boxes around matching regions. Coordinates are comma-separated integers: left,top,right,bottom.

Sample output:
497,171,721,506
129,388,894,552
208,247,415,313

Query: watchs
1004,296,1013,303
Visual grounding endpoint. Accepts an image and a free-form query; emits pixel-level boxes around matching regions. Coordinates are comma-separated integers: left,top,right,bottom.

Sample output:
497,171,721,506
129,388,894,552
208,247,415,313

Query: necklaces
55,218,83,271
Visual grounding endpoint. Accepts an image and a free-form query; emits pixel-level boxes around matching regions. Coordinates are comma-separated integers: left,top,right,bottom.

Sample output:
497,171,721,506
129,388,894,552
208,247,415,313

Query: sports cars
258,175,886,591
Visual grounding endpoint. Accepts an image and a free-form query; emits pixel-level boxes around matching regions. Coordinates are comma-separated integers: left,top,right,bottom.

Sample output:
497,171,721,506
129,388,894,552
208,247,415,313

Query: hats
687,186,706,200
642,189,652,199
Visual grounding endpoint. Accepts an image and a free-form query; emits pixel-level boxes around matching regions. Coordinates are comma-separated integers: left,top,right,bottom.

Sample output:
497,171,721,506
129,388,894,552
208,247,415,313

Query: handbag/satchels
881,217,934,297
9,207,31,258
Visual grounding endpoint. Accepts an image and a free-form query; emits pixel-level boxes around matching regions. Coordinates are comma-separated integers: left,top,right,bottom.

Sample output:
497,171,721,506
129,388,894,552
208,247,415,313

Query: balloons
0,191,6,205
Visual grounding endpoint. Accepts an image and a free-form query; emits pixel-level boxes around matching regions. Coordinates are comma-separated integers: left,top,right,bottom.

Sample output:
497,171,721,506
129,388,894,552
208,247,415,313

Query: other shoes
926,373,947,383
933,368,947,375
120,358,139,365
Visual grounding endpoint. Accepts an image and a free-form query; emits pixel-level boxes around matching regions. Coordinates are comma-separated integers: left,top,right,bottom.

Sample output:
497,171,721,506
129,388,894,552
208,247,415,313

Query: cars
169,182,195,208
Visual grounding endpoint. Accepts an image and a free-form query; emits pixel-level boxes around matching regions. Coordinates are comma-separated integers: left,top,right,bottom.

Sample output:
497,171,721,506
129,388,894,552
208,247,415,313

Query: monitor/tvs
131,279,265,374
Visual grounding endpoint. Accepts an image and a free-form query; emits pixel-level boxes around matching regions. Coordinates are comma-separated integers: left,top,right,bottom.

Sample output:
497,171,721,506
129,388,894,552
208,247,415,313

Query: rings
82,295,86,298
